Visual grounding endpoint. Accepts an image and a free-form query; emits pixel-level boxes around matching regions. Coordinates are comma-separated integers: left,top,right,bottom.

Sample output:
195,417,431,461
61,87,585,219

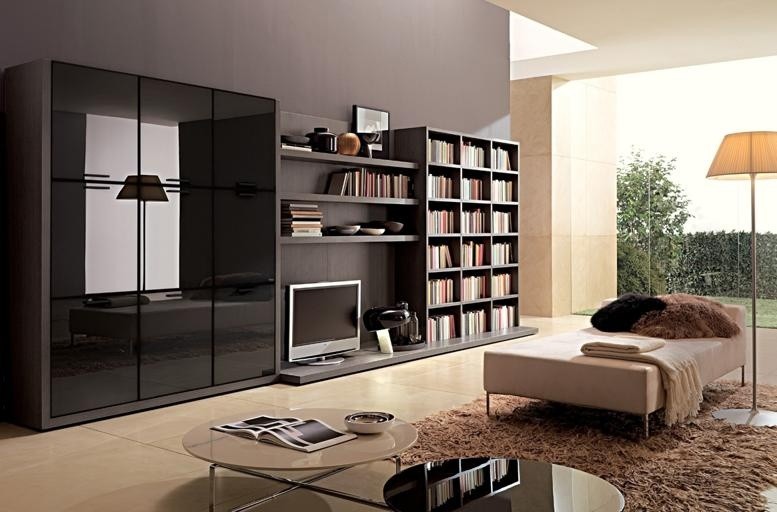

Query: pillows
590,294,666,333
217,275,272,302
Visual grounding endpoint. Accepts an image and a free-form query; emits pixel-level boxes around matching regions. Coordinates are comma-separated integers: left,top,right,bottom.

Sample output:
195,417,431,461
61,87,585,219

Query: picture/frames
353,105,390,159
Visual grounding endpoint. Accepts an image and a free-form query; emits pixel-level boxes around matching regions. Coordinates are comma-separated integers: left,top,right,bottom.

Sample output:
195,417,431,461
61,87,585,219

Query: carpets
50,328,275,380
393,378,777,512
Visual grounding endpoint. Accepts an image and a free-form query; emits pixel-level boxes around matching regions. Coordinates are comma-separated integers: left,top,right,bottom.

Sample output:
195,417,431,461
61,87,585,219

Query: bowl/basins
335,225,361,235
344,411,396,435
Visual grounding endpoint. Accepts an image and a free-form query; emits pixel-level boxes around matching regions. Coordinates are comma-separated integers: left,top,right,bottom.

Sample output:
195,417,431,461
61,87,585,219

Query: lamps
706,132,776,427
114,176,169,291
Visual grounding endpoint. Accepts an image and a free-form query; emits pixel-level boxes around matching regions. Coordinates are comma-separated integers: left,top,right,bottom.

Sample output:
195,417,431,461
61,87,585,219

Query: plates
281,135,311,144
359,227,386,236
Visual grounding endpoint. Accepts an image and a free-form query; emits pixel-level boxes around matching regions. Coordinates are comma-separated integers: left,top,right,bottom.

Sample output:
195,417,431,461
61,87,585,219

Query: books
427,244,457,269
463,140,488,168
325,168,414,198
493,305,518,332
464,275,488,302
208,413,359,453
427,315,458,343
280,200,325,239
493,240,515,267
492,271,513,298
493,211,514,234
493,178,515,202
428,138,455,165
493,146,513,171
462,309,488,337
463,239,486,268
281,144,313,154
462,176,484,201
427,278,457,305
462,207,488,236
429,209,457,235
427,459,510,511
428,171,455,201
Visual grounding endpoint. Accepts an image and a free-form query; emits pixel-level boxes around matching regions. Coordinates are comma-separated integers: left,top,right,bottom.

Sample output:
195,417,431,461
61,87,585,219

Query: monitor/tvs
288,280,362,366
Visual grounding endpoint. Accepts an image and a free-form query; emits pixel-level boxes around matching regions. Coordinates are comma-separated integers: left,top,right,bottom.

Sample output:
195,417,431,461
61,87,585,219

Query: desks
183,406,419,512
384,456,624,512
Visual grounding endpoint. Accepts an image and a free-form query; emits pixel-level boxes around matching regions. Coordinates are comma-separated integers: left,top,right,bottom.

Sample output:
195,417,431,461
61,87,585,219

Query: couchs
485,294,748,436
67,277,274,356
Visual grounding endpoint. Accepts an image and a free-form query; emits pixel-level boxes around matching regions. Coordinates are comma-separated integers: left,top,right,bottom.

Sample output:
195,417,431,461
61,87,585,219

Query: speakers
363,307,411,332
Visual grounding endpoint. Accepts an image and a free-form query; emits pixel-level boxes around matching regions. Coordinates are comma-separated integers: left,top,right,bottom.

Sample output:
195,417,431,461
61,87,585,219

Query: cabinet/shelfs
139,76,212,411
0,59,139,431
280,144,425,386
213,89,281,397
394,127,538,354
